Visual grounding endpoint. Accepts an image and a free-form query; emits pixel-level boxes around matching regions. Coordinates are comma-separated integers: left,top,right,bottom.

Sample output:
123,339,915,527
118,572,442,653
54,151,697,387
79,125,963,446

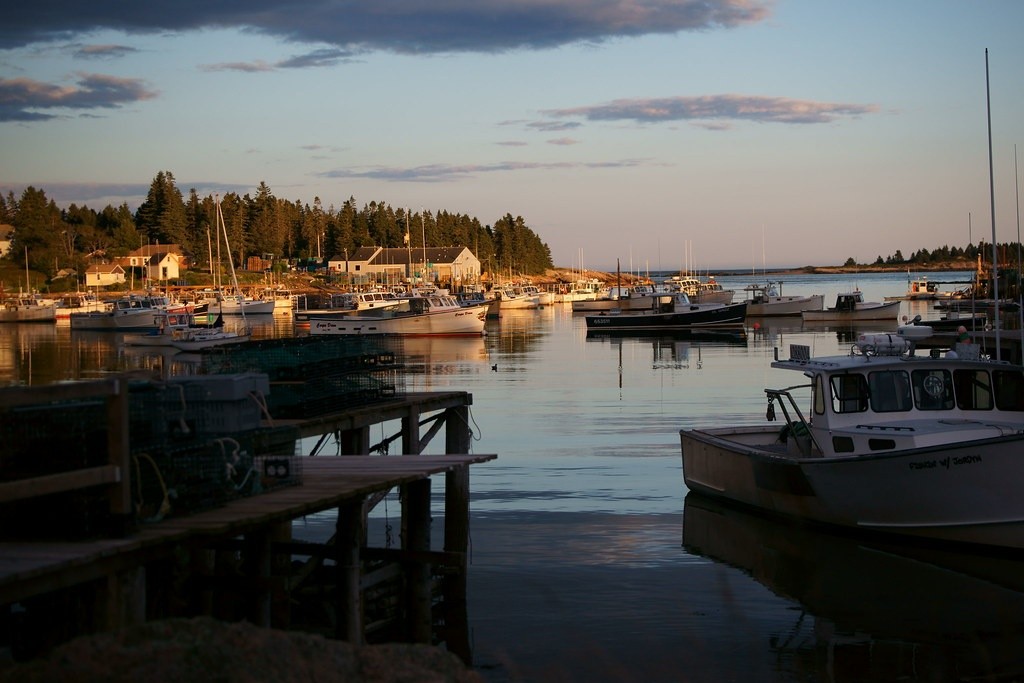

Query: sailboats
1,46,1023,406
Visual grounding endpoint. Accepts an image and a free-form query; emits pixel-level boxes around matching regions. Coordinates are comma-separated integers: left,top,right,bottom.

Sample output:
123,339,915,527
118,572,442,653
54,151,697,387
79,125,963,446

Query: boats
679,486,1024,683
673,329,1024,559
